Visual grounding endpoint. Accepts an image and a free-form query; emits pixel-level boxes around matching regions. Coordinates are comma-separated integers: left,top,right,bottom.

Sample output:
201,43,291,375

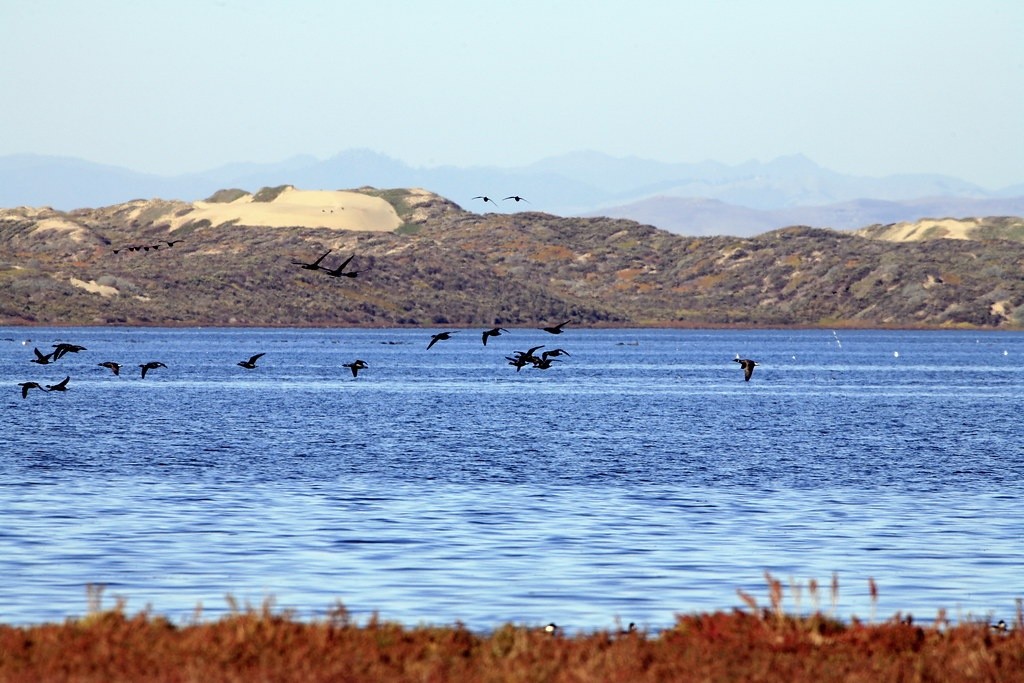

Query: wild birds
15,193,759,400
